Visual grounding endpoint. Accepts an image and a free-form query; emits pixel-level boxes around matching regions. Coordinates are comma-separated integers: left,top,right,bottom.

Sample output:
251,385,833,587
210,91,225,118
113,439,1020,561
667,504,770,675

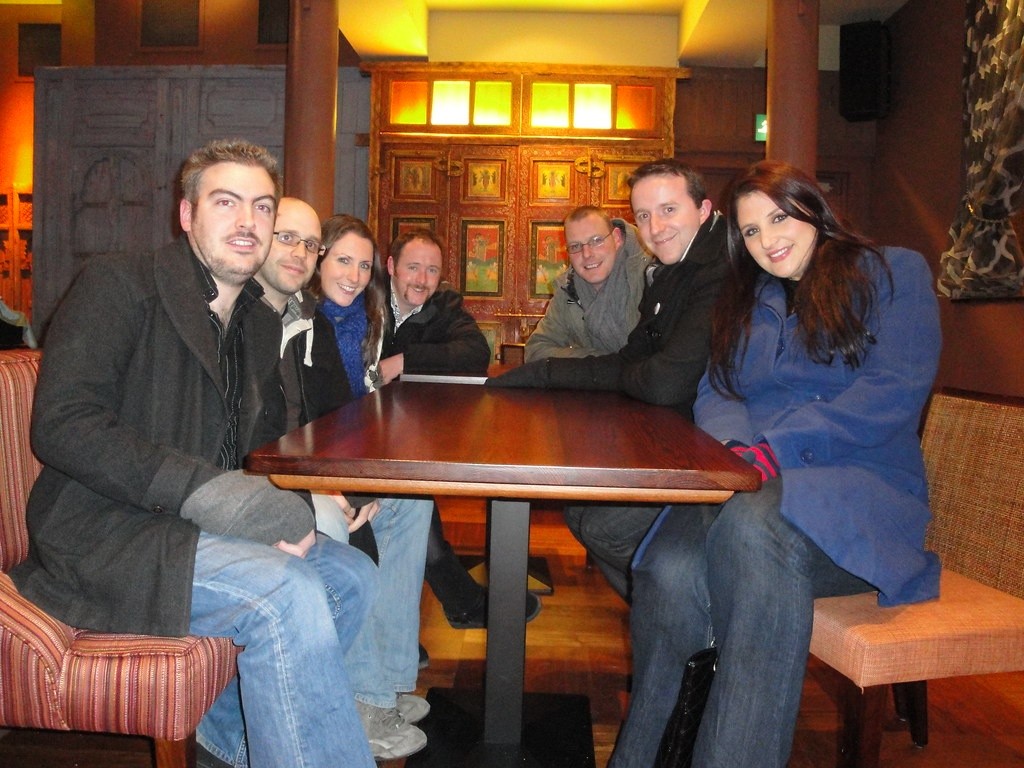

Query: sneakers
355,694,431,761
445,584,542,629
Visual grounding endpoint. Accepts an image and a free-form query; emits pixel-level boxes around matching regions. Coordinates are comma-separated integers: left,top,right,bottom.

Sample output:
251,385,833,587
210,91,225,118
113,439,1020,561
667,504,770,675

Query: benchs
0,345,240,766
808,390,1024,768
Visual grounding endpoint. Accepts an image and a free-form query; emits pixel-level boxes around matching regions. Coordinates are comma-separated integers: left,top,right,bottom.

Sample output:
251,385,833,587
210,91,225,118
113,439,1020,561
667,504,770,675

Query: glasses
274,231,326,257
565,228,613,254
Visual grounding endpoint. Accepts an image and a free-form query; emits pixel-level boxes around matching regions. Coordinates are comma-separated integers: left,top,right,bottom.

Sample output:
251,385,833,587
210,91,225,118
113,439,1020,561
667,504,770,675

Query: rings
376,372,378,376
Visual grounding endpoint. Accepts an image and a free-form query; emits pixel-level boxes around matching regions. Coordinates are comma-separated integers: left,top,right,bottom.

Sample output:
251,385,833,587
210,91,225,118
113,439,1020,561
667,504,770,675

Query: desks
247,378,761,768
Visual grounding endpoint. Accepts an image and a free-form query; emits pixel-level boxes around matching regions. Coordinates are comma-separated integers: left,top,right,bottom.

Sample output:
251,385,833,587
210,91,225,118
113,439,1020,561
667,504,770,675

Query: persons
599,158,941,768
523,202,661,363
8,137,377,768
366,226,492,378
250,193,434,759
301,212,542,668
486,158,751,561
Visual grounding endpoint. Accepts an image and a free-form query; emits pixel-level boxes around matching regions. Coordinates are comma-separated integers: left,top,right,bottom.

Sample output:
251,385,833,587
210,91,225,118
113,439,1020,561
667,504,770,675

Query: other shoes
418,643,430,669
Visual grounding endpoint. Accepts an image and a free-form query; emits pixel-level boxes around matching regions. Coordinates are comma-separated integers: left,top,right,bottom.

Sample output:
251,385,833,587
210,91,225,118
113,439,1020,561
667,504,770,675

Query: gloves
724,439,781,482
